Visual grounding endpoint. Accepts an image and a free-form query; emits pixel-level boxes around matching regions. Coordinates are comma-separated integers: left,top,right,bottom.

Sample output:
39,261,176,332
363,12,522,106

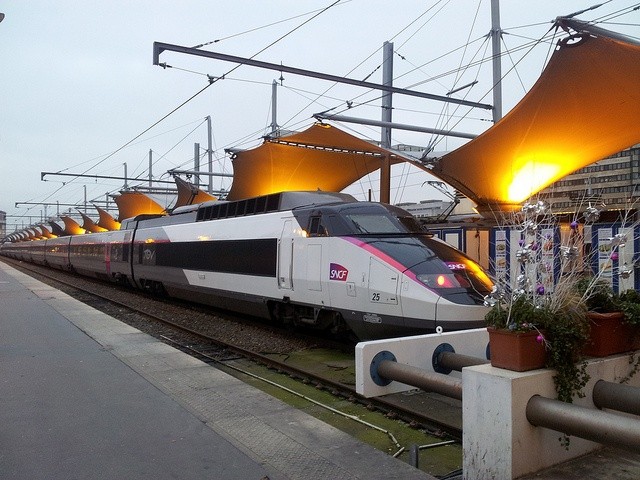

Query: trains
1,186,504,345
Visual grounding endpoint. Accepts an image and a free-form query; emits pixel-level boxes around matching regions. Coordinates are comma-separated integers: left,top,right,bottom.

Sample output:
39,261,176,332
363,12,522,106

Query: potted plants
484,293,591,453
573,275,640,384
